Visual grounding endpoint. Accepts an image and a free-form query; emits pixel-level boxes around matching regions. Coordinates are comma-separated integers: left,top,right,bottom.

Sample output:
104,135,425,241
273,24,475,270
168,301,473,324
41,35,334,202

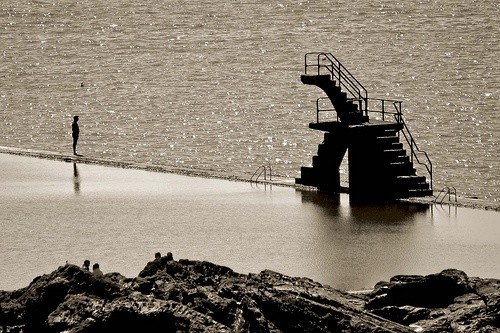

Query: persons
92,263,103,275
167,252,173,261
153,253,161,260
81,260,90,270
72,116,80,155
